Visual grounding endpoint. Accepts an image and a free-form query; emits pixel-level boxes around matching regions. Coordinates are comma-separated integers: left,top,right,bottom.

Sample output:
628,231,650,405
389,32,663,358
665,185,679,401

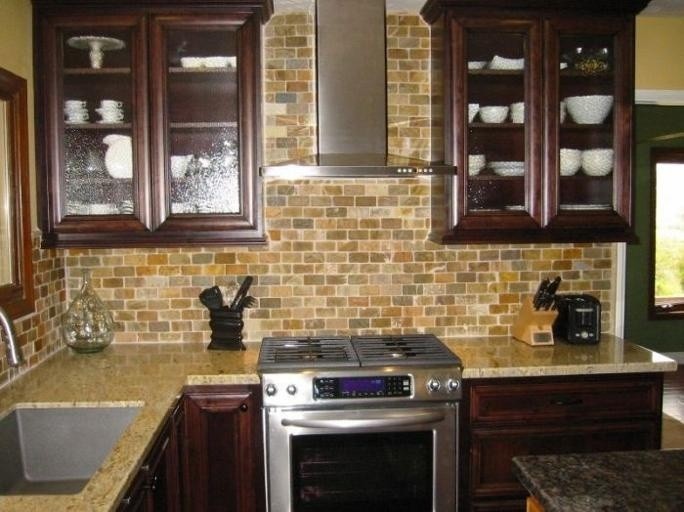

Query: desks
509,449,683,510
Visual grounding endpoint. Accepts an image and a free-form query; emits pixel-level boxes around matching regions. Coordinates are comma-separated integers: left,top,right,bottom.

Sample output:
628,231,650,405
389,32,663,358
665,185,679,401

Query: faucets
0,306,26,368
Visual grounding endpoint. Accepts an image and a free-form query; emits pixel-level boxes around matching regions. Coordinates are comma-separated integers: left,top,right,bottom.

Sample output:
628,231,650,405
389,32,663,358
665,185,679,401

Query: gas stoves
257,333,462,400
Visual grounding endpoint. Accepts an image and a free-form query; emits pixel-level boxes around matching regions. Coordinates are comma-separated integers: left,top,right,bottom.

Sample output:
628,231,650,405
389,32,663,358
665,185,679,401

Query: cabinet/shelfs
186,390,267,511
420,0,651,244
29,2,274,249
461,372,666,510
115,396,186,511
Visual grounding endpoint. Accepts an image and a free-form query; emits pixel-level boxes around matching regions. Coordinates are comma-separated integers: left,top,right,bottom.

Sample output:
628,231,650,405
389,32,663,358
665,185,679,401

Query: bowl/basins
181,57,236,68
557,144,615,179
468,102,564,125
467,61,486,69
564,93,615,125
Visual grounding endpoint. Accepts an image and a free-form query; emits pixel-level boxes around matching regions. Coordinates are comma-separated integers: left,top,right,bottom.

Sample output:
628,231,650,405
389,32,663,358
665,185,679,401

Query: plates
560,62,568,70
486,54,524,69
502,202,610,213
467,153,487,177
468,208,499,212
490,159,524,178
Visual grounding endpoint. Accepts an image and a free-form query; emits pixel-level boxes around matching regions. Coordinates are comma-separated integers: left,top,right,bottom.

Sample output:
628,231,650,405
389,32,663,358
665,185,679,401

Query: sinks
0,398,147,496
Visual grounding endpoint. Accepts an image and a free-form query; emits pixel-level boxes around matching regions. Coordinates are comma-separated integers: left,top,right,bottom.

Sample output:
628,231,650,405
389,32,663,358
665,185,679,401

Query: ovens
263,401,460,511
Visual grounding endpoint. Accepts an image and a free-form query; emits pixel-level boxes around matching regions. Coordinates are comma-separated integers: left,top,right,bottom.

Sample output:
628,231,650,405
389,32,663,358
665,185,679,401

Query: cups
65,98,124,125
104,135,213,178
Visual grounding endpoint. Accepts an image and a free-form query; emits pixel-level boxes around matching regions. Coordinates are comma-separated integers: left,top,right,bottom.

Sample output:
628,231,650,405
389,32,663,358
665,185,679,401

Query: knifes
533,274,561,312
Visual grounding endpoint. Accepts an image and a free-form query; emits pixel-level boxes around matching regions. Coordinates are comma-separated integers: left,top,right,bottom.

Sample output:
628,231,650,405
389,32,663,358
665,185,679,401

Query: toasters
554,293,601,345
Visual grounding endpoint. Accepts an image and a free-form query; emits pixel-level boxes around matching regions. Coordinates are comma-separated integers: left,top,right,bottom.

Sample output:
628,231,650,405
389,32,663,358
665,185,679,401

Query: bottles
62,269,115,354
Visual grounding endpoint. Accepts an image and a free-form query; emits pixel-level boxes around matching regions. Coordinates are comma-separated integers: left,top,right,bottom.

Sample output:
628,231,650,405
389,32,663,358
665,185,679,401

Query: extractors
255,1,458,180
